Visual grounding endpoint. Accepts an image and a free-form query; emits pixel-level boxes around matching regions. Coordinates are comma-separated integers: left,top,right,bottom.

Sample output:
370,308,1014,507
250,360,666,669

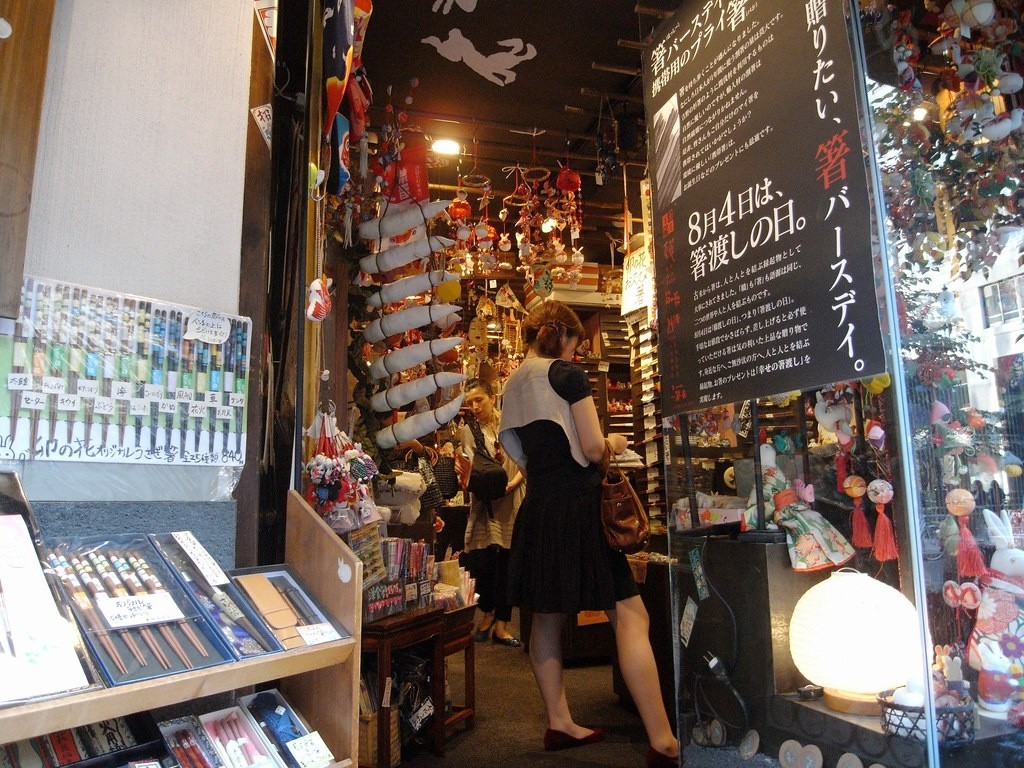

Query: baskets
876,686,975,749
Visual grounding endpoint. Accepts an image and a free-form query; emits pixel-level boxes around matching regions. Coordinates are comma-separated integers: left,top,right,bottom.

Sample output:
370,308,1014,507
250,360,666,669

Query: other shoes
544,727,606,751
475,617,495,642
492,629,521,647
646,746,679,768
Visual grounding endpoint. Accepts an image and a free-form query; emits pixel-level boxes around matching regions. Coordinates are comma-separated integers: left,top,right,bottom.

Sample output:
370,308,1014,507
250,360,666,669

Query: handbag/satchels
600,438,650,555
405,447,459,499
405,450,443,512
466,420,508,519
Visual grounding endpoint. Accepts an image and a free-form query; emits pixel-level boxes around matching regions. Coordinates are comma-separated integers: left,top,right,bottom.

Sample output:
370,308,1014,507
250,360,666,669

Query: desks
361,604,475,768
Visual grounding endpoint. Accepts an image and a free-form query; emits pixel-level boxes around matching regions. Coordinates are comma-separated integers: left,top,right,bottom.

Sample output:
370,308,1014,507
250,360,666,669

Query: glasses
494,441,503,462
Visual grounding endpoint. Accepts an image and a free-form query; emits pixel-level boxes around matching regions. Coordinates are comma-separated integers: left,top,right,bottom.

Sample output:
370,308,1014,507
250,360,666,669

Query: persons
461,379,527,646
499,301,687,768
747,445,855,572
379,402,437,557
971,479,1004,510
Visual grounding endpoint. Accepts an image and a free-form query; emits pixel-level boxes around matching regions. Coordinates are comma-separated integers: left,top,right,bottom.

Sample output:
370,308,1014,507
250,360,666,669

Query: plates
675,435,698,446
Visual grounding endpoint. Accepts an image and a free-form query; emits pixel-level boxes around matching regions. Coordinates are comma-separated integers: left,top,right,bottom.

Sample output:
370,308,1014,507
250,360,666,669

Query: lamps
789,567,933,716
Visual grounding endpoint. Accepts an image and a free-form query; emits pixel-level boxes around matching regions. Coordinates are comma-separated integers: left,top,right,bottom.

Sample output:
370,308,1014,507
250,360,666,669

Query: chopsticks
572,362,602,410
168,704,295,768
629,327,667,535
362,536,430,624
756,401,797,444
10,279,248,463
284,585,323,624
186,544,273,651
45,547,209,675
601,320,630,359
